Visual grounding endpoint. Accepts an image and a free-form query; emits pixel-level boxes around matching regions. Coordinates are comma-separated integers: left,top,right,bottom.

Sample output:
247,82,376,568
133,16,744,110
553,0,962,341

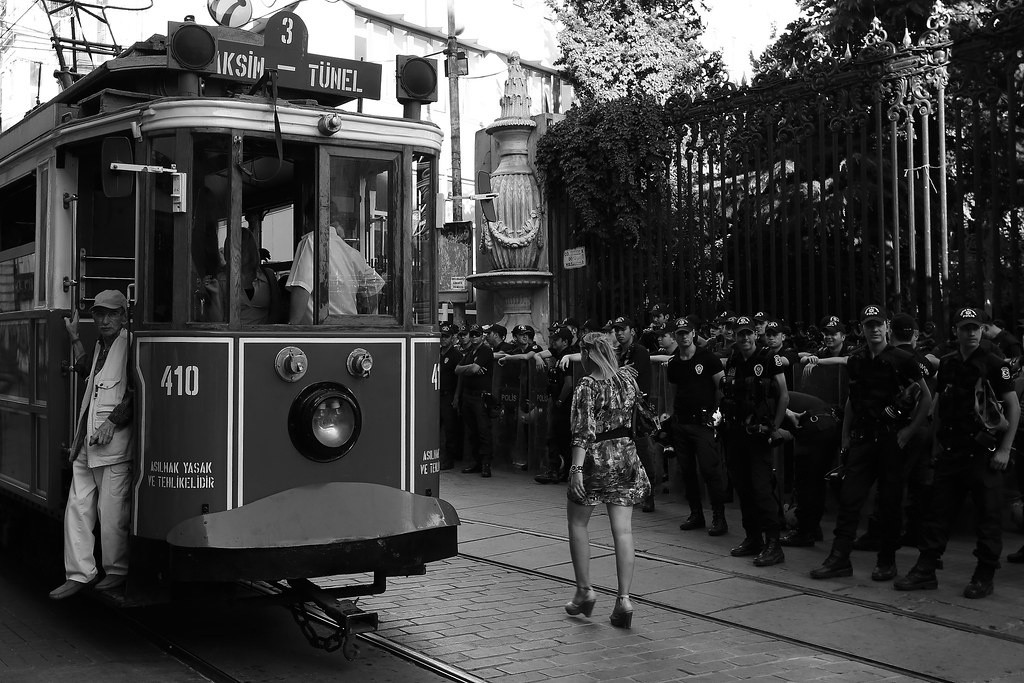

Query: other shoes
49,575,99,599
461,454,492,477
901,524,920,546
95,575,123,590
852,530,882,551
1007,546,1024,563
440,455,455,470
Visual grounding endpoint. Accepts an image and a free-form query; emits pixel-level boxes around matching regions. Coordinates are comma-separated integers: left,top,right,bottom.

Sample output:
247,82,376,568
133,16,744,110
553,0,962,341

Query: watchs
571,465,583,472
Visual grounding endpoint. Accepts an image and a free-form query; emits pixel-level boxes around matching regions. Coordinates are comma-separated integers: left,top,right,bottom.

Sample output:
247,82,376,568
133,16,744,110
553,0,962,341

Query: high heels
565,585,597,618
609,594,634,628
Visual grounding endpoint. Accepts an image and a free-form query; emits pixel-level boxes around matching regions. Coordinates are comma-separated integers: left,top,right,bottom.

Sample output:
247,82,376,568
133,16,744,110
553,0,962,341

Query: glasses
92,310,125,320
766,332,777,337
825,331,836,336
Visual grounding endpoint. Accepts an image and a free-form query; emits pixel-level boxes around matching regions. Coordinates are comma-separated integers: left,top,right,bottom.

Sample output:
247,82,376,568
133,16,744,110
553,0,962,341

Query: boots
730,529,764,556
873,559,898,580
778,514,823,547
809,549,853,578
642,484,655,513
752,533,784,565
535,465,570,484
964,560,997,599
709,505,728,535
894,551,942,590
680,504,706,530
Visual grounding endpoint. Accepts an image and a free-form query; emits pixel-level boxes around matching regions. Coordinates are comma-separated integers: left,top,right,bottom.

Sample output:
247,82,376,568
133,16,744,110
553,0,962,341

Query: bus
0,0,463,664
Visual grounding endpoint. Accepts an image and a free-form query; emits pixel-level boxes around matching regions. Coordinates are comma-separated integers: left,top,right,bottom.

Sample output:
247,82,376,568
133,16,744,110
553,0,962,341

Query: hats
440,305,1024,339
89,289,127,311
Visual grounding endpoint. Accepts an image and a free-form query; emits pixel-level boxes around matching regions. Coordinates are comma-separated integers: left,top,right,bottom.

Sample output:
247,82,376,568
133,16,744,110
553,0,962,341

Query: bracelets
71,338,80,345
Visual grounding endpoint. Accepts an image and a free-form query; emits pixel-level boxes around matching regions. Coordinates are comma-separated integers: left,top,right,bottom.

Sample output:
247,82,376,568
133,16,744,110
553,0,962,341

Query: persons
440,318,603,484
566,332,651,629
50,291,132,598
202,227,284,323
602,304,1024,597
285,201,384,325
